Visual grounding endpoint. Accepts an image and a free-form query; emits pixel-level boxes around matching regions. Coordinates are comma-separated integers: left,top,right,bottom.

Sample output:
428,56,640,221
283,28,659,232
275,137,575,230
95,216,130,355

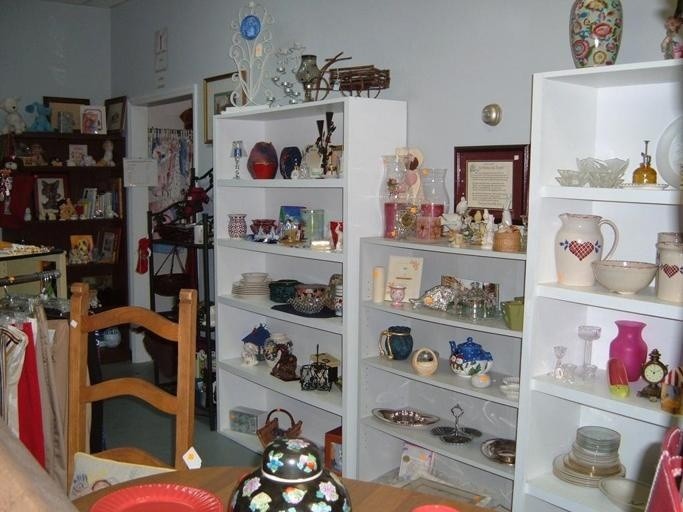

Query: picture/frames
204,69,248,146
42,97,129,134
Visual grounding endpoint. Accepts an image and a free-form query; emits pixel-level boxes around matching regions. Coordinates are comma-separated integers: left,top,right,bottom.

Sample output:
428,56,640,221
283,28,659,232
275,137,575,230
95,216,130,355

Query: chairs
63,283,199,498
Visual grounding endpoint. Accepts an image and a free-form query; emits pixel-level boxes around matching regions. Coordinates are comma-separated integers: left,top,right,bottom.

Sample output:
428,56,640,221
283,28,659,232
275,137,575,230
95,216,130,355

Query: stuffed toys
98,142,116,168
0,95,25,136
24,101,54,134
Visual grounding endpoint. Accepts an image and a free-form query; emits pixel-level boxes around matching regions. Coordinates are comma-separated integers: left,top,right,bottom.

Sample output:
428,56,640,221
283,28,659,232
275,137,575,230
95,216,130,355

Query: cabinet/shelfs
0,133,134,365
358,236,525,512
149,199,217,431
210,95,408,480
513,59,682,511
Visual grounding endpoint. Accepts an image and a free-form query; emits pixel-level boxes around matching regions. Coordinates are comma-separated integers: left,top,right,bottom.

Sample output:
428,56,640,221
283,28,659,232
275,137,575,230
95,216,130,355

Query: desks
0,239,64,297
74,469,489,508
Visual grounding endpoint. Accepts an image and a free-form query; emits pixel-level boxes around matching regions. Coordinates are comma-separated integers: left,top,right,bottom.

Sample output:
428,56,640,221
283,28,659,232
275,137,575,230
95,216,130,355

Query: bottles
372,265,384,303
377,153,450,240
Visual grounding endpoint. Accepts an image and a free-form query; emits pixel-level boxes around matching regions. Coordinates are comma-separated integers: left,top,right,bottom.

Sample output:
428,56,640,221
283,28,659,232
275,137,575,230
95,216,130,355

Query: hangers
0,270,69,330
149,126,193,149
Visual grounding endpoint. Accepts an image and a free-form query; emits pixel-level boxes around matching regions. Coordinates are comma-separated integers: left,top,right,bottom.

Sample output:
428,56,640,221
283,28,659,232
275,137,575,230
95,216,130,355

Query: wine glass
388,285,406,306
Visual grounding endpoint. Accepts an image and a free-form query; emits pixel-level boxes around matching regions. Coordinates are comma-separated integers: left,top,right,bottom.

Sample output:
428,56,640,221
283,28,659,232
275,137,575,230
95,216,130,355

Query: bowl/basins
553,156,630,189
240,272,268,284
589,258,660,297
596,476,651,512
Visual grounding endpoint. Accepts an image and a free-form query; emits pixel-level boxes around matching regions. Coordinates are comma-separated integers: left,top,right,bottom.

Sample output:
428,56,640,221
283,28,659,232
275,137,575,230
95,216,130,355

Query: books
79,186,113,220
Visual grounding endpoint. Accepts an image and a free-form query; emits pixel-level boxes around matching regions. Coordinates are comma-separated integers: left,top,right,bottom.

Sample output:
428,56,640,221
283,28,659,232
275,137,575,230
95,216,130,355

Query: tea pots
447,336,493,380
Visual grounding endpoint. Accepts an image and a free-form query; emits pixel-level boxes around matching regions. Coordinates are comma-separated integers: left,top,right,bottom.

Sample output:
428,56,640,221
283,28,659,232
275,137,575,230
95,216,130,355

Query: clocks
637,348,669,399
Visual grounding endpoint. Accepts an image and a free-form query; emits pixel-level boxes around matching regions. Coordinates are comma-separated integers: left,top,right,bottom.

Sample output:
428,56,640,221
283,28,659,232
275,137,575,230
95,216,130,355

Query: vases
567,1,623,69
609,319,649,383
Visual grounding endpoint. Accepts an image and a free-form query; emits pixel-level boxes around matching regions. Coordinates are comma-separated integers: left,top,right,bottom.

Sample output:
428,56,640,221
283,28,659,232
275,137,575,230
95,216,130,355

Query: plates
654,113,683,189
618,182,670,191
480,437,515,464
550,426,627,490
371,406,439,428
231,277,273,299
86,482,222,511
